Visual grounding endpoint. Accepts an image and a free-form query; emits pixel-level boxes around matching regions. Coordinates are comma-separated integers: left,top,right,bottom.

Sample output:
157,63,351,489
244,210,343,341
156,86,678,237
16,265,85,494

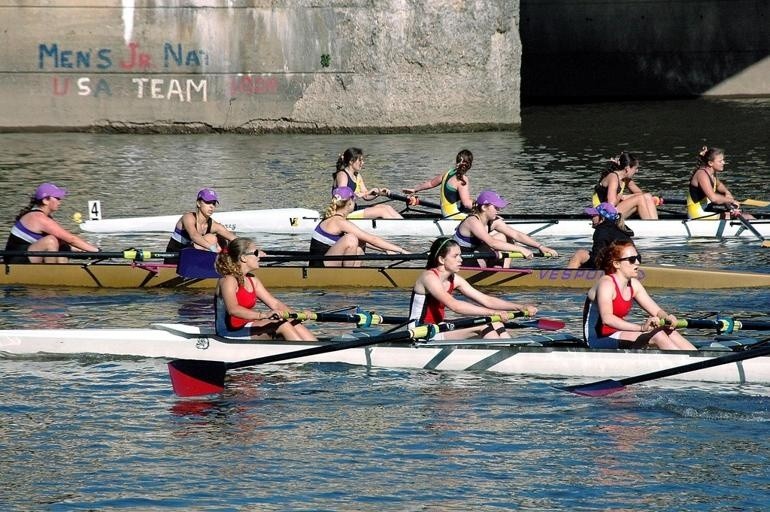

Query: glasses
616,255,641,264
243,249,258,257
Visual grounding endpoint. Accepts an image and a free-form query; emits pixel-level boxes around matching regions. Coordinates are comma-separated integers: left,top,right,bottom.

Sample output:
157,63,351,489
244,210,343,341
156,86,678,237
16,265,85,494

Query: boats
0,248,770,293
74,207,770,239
1,321,769,386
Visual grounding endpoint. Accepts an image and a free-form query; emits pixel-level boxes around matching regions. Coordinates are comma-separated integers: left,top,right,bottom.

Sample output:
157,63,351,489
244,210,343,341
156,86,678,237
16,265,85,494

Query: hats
584,202,617,221
476,191,512,209
197,188,220,205
333,186,358,200
35,183,69,200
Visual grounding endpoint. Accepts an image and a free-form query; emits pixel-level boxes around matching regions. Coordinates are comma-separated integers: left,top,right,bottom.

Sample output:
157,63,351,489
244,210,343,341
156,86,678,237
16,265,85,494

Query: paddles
659,196,769,208
167,306,536,396
550,339,766,398
176,248,552,279
272,312,565,333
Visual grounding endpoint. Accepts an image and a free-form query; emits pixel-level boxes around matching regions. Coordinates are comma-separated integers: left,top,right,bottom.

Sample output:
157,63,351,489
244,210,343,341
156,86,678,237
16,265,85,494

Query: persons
163,187,266,265
686,146,758,222
309,186,411,268
2,183,100,263
214,237,318,341
566,202,641,271
326,149,406,219
453,191,558,268
583,236,699,351
591,149,661,220
408,238,536,340
403,150,473,221
